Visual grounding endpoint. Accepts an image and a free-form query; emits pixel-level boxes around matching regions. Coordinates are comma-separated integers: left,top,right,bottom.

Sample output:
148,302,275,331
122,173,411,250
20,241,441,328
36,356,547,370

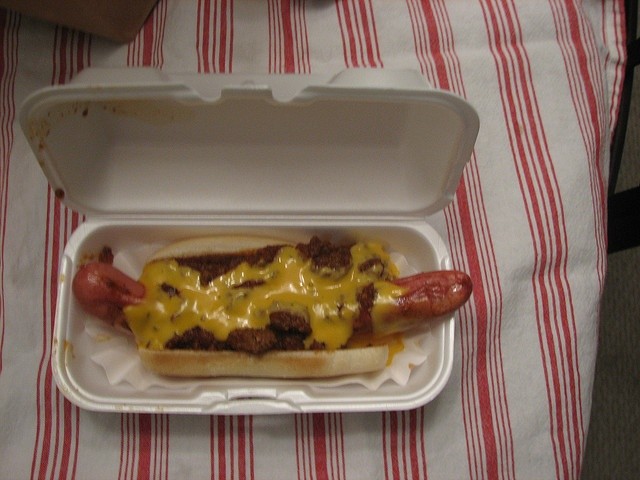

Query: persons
1,0,159,44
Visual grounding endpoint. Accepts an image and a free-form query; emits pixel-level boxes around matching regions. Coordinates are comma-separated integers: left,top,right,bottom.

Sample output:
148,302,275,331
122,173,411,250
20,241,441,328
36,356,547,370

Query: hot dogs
71,234,473,380
